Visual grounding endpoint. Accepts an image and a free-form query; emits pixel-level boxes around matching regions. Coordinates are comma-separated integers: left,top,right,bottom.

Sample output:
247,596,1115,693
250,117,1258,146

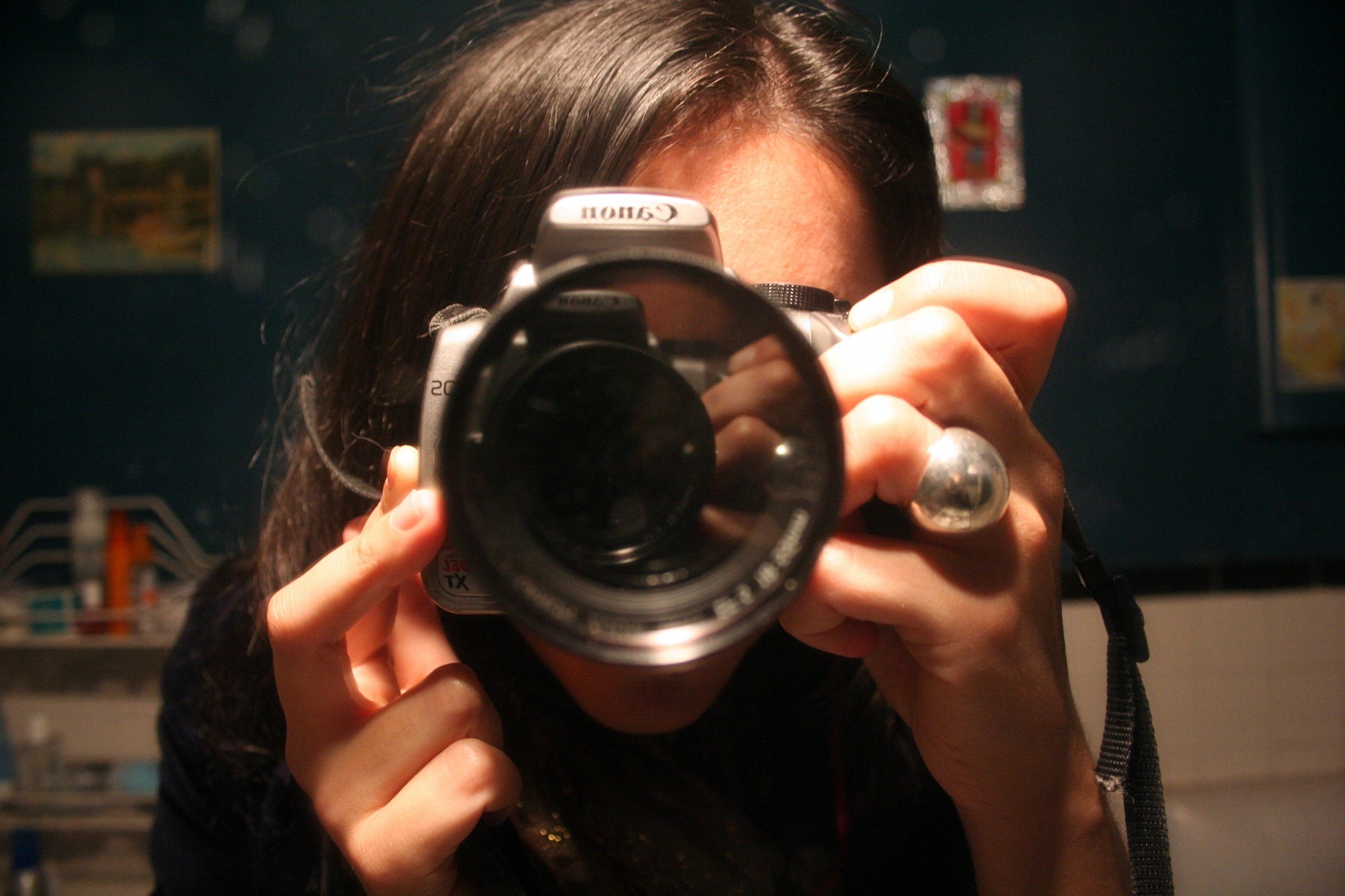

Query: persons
148,0,1174,896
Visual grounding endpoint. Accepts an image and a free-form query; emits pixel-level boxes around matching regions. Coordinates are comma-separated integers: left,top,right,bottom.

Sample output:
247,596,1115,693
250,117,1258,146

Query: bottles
71,492,106,635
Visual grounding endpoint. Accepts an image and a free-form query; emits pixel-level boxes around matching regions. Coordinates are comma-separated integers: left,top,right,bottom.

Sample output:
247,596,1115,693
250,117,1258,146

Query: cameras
417,187,904,676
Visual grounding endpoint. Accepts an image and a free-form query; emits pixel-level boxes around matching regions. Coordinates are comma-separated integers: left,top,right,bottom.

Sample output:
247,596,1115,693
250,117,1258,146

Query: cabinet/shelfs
0,569,193,896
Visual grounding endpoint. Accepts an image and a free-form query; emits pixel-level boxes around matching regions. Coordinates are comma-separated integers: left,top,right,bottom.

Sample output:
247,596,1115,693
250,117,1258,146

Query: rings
908,427,1012,535
